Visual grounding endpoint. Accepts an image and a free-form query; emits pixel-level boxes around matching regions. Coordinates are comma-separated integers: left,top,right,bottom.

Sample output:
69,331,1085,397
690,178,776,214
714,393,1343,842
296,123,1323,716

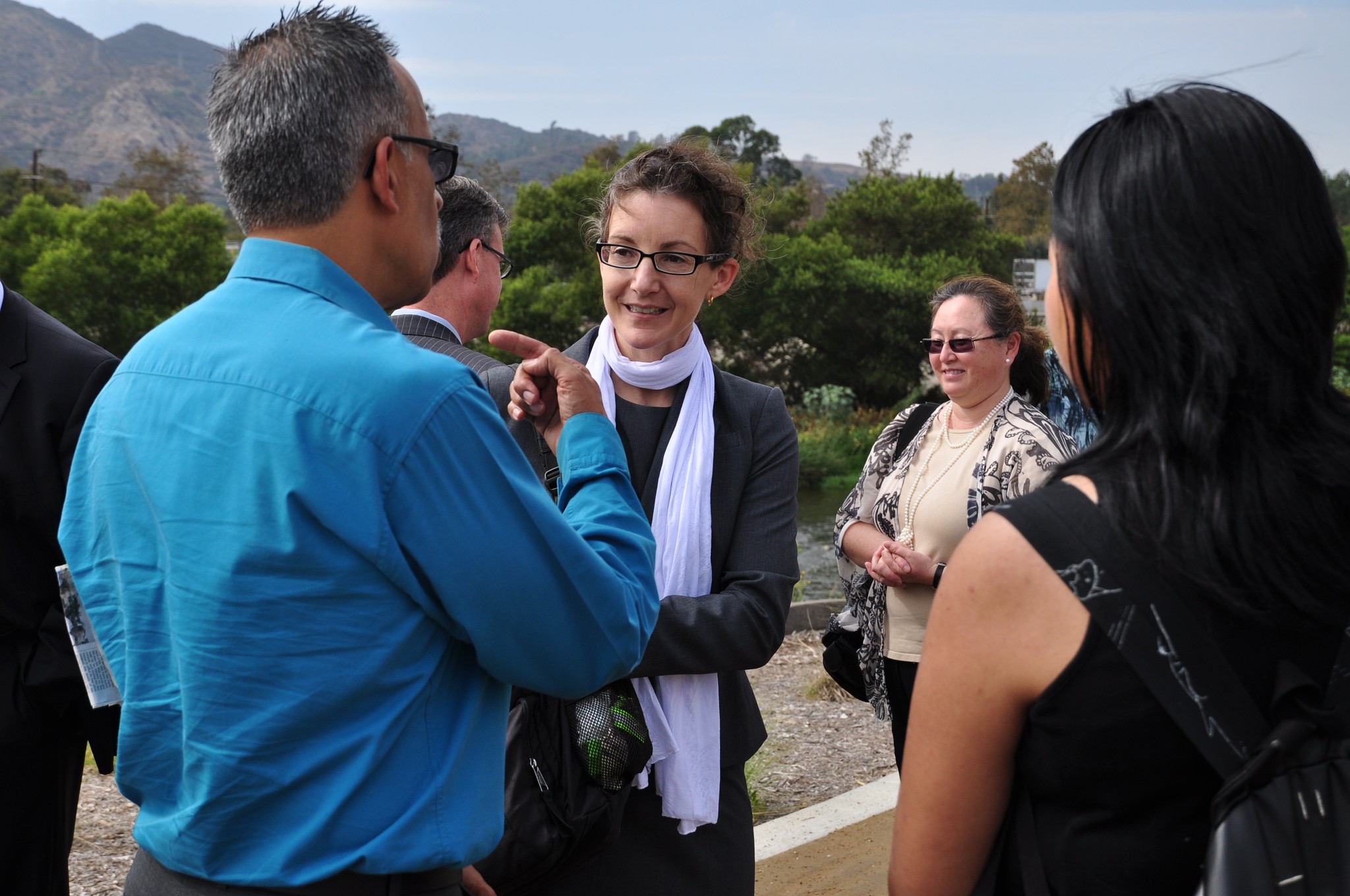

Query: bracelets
930,562,946,591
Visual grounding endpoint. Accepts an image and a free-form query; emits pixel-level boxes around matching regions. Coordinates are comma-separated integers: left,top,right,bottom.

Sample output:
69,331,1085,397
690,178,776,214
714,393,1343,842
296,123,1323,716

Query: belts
267,867,462,896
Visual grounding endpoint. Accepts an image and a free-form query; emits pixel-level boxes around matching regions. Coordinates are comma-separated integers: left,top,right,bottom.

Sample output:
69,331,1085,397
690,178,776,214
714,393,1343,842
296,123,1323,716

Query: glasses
921,331,1012,354
457,240,515,278
595,238,729,276
364,134,459,185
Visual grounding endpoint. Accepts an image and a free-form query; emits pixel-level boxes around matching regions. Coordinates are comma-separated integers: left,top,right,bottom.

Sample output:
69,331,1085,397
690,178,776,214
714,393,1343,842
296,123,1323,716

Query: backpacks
987,487,1350,896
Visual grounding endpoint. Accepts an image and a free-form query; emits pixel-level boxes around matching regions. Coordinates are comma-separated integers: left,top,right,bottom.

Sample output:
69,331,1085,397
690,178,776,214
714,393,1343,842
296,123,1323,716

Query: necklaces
896,387,1014,550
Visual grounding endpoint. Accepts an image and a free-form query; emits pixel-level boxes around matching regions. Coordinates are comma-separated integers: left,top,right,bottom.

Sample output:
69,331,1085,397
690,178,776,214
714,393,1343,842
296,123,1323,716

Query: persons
888,77,1350,896
386,175,514,374
54,1,659,896
0,289,123,896
833,277,1076,790
477,146,800,896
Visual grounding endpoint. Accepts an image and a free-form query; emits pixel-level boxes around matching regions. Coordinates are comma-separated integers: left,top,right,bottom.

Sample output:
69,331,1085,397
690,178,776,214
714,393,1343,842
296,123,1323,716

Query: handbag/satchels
821,624,870,702
485,679,653,888
